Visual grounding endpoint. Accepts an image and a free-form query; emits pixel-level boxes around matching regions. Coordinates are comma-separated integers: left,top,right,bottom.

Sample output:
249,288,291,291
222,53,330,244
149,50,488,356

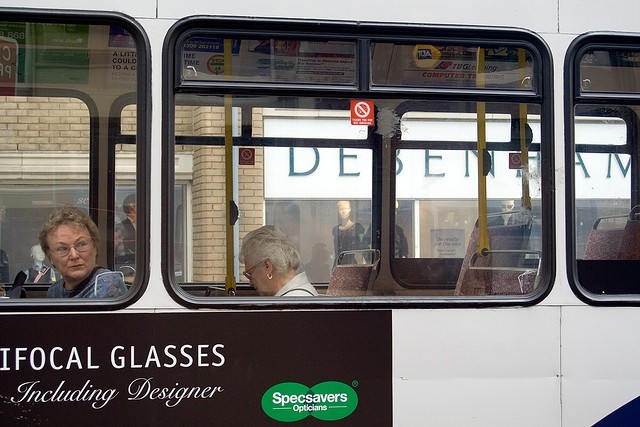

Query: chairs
326,250,381,296
618,206,640,259
9,271,27,299
585,215,628,260
454,249,544,295
205,285,236,296
455,212,533,294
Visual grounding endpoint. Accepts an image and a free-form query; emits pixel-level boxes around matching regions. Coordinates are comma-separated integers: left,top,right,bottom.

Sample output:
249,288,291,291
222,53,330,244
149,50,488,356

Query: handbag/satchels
6,271,28,298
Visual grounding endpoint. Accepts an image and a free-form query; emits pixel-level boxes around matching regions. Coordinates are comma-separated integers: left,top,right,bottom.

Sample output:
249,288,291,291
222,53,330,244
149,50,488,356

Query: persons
487,198,525,227
236,222,323,299
114,194,137,280
39,207,127,297
364,195,408,263
333,197,365,266
22,242,57,286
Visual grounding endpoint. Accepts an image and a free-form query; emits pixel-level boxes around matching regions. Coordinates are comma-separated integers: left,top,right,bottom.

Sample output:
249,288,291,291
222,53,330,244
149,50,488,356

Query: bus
0,0,640,427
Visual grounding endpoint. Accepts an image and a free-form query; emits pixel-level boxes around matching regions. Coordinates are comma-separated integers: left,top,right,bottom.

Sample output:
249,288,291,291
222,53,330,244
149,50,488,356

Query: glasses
46,236,94,257
242,261,266,280
